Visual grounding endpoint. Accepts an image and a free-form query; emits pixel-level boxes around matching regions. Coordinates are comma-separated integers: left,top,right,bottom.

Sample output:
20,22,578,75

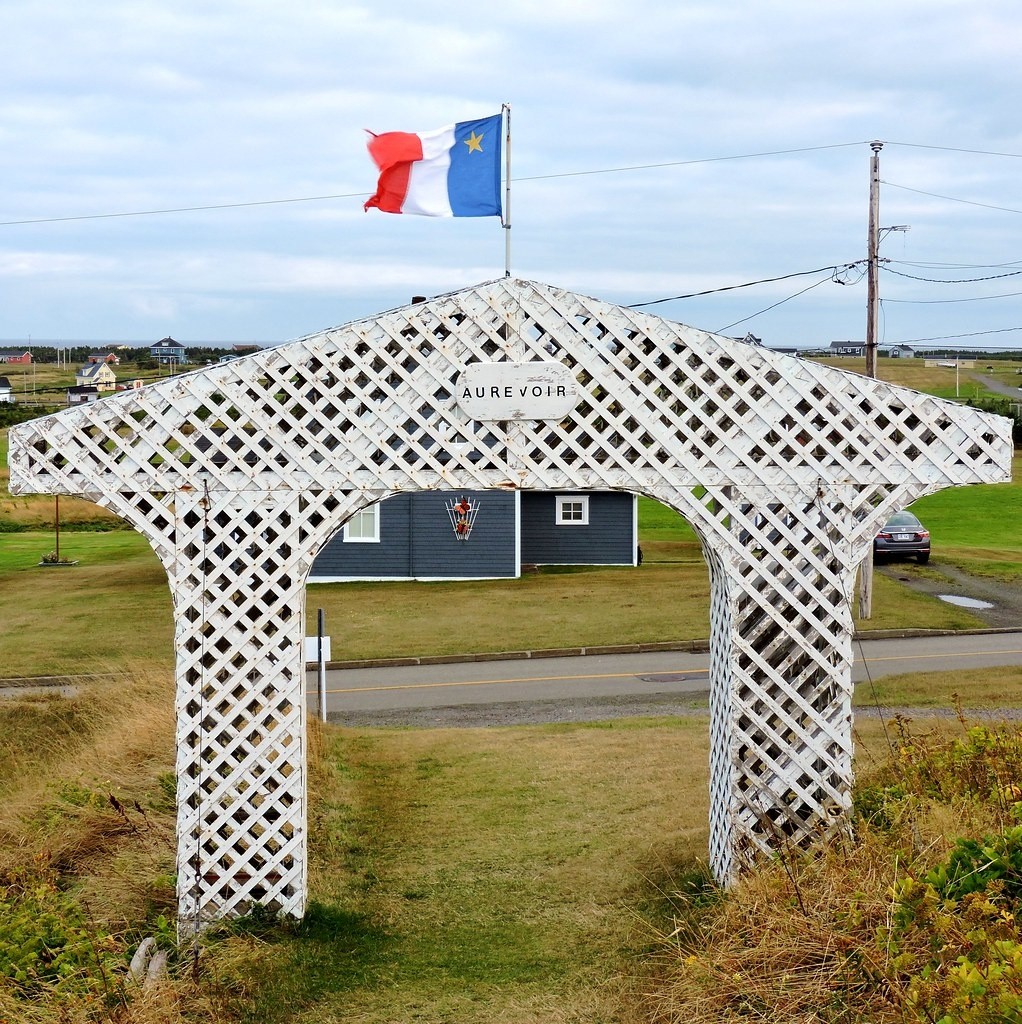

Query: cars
857,510,931,566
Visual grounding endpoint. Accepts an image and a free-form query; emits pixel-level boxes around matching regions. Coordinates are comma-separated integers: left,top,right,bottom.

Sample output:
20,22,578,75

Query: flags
361,113,502,217
